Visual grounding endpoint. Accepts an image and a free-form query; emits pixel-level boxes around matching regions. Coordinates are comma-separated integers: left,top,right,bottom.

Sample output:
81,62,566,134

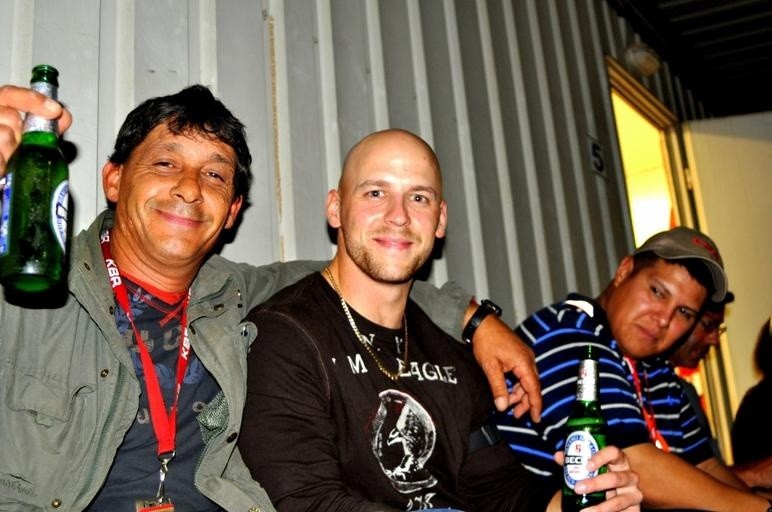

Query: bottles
562,345,607,511
3,64,68,309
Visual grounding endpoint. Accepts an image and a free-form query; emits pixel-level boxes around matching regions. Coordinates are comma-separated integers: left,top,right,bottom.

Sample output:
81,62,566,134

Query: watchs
463,298,503,343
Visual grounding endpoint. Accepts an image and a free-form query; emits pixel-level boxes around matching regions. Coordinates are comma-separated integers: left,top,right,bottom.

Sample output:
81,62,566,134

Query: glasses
698,318,728,337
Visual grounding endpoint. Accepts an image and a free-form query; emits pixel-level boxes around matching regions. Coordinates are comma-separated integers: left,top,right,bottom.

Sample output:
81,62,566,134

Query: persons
240,126,643,512
665,291,772,489
487,225,772,512
1,81,542,512
732,317,772,463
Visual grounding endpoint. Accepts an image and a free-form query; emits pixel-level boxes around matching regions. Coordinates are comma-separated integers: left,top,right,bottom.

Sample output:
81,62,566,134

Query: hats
632,227,734,304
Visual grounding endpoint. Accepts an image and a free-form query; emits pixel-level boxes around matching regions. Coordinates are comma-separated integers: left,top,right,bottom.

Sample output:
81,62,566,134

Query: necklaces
322,262,410,384
